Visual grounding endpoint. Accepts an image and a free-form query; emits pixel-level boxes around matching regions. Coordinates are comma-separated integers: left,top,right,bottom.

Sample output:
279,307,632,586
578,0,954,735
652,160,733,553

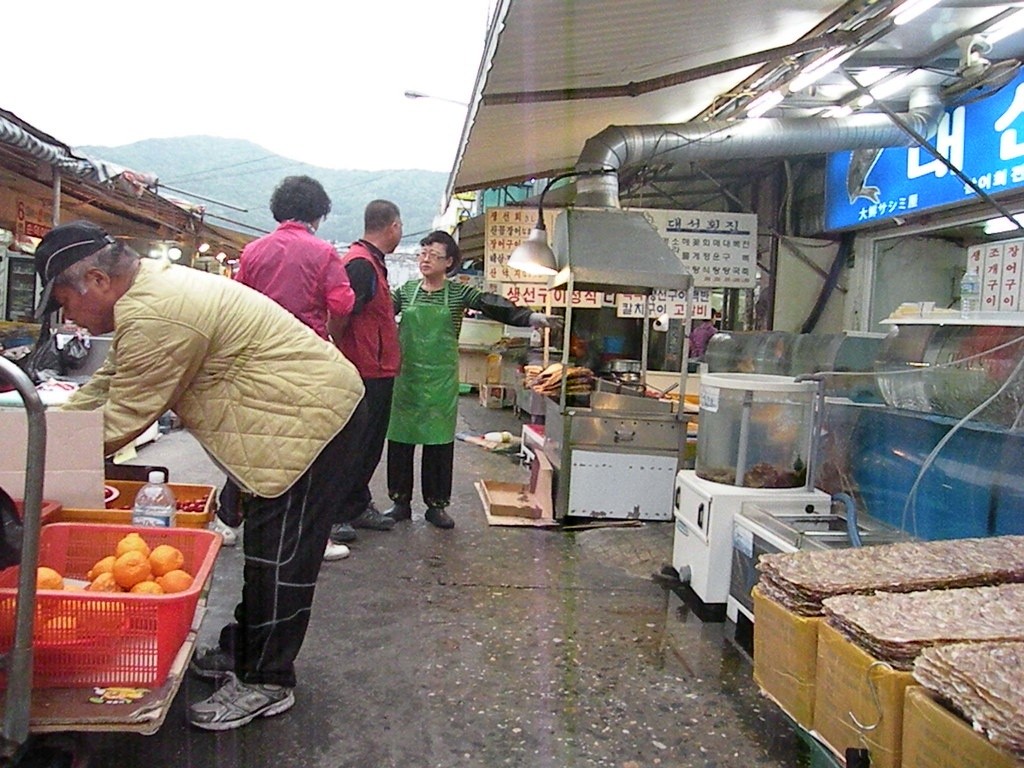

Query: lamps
198,241,210,253
507,166,617,276
167,243,183,260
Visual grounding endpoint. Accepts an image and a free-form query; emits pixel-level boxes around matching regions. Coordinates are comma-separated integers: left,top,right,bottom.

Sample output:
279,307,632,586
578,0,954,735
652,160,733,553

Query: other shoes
208,514,236,546
381,502,413,523
425,507,455,529
323,543,350,562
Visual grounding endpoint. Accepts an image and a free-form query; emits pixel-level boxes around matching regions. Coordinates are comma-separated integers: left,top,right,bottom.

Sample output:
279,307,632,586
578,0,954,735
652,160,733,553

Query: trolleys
1,356,216,768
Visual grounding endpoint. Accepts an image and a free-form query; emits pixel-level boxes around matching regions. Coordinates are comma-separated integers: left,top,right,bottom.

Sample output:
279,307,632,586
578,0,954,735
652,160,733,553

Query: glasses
416,252,449,260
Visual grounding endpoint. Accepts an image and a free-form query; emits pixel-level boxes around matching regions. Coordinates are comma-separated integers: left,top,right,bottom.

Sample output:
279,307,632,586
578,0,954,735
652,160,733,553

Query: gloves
530,313,563,330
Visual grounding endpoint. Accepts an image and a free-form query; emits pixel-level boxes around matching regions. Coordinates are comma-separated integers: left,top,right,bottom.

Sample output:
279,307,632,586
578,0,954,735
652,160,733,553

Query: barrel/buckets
596,335,626,367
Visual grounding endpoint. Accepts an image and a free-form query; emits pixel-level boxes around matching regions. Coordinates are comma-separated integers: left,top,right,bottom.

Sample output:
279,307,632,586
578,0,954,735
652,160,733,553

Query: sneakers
351,503,394,529
330,523,356,541
191,645,236,677
188,678,295,729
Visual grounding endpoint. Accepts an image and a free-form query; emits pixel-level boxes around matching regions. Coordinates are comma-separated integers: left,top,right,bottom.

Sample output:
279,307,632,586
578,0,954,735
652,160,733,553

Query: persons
689,309,719,358
380,231,566,529
212,173,355,561
34,219,367,730
330,200,403,543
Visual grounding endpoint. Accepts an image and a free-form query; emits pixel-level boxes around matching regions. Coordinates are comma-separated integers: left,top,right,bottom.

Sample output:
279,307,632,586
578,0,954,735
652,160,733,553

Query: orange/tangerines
0,533,194,643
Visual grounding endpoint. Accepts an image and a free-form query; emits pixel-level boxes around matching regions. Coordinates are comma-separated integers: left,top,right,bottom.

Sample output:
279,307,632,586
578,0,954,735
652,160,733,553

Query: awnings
446,0,904,206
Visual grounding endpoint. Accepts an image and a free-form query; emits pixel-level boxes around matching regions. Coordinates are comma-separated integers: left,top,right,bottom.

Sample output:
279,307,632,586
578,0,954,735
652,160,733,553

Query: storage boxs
0,408,225,691
479,383,515,408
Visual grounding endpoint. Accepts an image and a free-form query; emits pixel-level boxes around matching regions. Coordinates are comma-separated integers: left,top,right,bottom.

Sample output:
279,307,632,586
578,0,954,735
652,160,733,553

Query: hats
34,219,116,318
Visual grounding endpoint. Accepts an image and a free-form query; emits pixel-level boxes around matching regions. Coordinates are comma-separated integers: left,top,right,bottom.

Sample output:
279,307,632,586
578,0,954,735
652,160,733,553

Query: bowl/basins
105,484,121,508
459,384,472,393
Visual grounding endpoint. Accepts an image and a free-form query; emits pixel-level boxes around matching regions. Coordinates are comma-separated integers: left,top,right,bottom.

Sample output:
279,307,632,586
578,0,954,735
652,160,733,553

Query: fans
940,33,1021,105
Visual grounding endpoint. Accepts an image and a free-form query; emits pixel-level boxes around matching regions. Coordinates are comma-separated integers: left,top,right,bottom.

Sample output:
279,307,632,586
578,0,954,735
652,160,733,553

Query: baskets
0,522,223,687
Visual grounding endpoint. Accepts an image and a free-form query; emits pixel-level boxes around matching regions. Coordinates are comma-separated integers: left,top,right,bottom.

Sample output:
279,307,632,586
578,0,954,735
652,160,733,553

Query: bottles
481,431,513,443
131,471,178,528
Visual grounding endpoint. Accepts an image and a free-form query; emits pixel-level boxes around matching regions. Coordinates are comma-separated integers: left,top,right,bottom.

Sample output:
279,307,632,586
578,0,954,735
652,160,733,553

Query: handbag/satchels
59,335,87,371
24,336,64,384
